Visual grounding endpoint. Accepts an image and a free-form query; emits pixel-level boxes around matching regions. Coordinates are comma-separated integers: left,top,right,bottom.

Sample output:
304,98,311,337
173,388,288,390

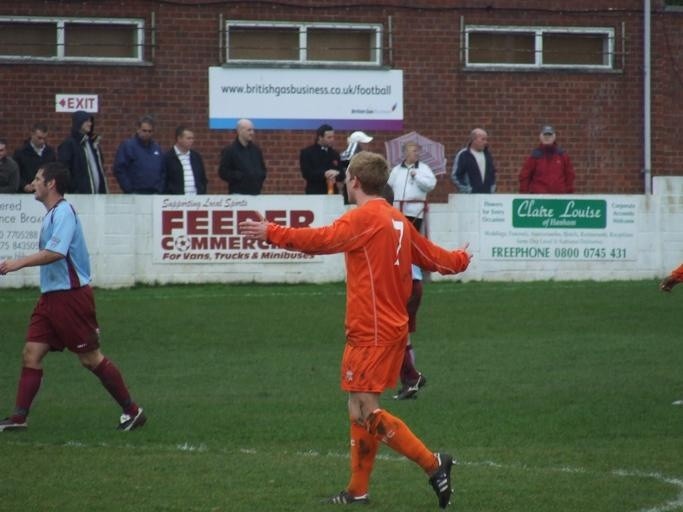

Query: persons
660,264,683,292
450,128,497,193
238,150,474,509
112,116,167,194
300,124,345,194
518,126,575,194
384,184,426,400
165,127,208,194
0,161,147,433
58,111,110,194
218,119,267,195
340,130,374,171
386,142,438,232
0,139,20,193
12,122,56,193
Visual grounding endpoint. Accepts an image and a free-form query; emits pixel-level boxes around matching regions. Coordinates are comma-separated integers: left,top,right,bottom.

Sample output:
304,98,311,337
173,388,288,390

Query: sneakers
324,490,372,507
115,406,147,434
426,452,455,510
0,415,29,433
392,372,426,401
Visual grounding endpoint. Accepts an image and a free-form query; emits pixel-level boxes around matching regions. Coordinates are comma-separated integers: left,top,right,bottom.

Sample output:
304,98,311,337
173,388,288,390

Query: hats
347,131,373,145
540,125,553,135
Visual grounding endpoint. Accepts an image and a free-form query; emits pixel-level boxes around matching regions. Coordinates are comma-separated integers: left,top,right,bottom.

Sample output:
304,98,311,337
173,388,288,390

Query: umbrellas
383,130,447,184
